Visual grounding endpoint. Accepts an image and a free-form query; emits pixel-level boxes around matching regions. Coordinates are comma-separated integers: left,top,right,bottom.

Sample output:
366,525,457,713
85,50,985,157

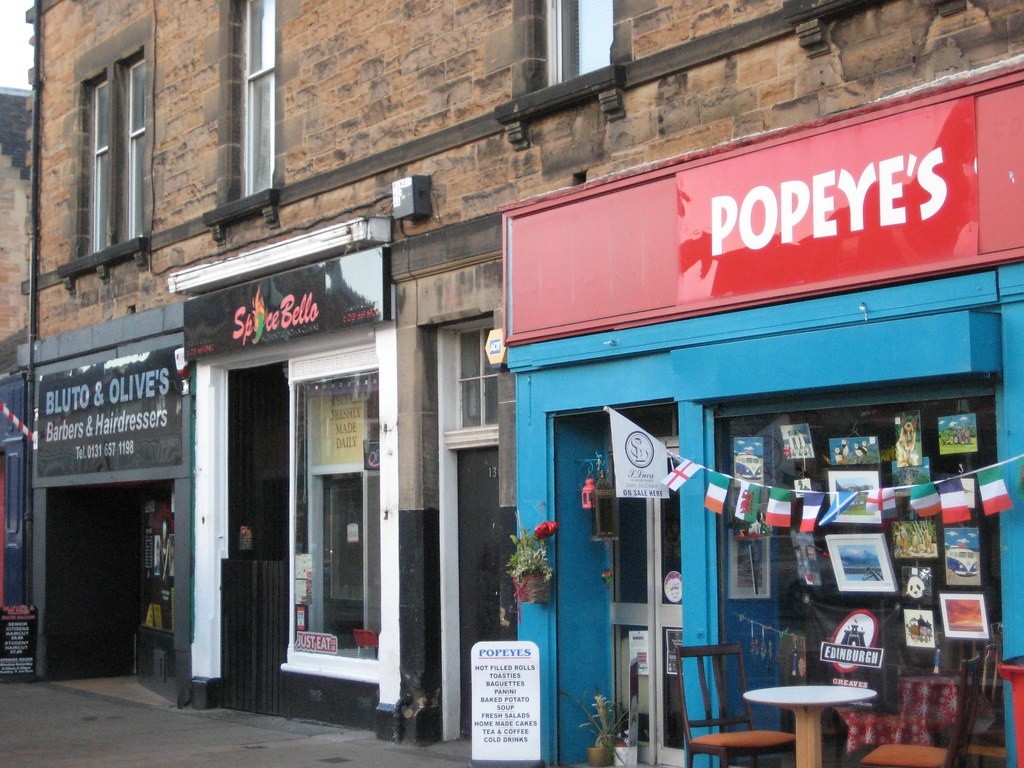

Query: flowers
617,730,636,747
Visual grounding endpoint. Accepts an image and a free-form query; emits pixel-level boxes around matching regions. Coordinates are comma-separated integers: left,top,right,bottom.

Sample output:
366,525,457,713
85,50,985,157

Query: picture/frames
727,529,772,601
938,590,992,641
824,533,898,592
826,469,882,526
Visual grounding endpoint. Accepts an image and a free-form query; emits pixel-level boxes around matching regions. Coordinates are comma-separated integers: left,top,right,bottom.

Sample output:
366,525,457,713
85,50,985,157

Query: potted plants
560,689,629,767
505,528,552,604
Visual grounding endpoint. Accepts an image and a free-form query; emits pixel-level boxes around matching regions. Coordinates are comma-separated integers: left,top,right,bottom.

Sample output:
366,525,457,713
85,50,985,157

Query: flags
609,404,1024,533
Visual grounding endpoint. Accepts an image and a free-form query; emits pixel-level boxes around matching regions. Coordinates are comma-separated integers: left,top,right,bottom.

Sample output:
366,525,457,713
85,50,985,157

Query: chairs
860,650,983,768
675,640,797,767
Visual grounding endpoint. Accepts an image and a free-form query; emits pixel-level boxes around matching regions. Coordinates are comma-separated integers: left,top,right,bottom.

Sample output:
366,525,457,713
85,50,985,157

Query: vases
615,739,627,747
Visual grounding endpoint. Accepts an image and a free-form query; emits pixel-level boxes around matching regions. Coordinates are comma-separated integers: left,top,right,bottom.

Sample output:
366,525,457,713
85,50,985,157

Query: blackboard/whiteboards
0,604,40,678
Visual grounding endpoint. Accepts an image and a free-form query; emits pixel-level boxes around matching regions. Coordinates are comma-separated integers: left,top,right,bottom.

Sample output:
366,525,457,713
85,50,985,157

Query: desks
896,675,1004,745
743,686,877,768
